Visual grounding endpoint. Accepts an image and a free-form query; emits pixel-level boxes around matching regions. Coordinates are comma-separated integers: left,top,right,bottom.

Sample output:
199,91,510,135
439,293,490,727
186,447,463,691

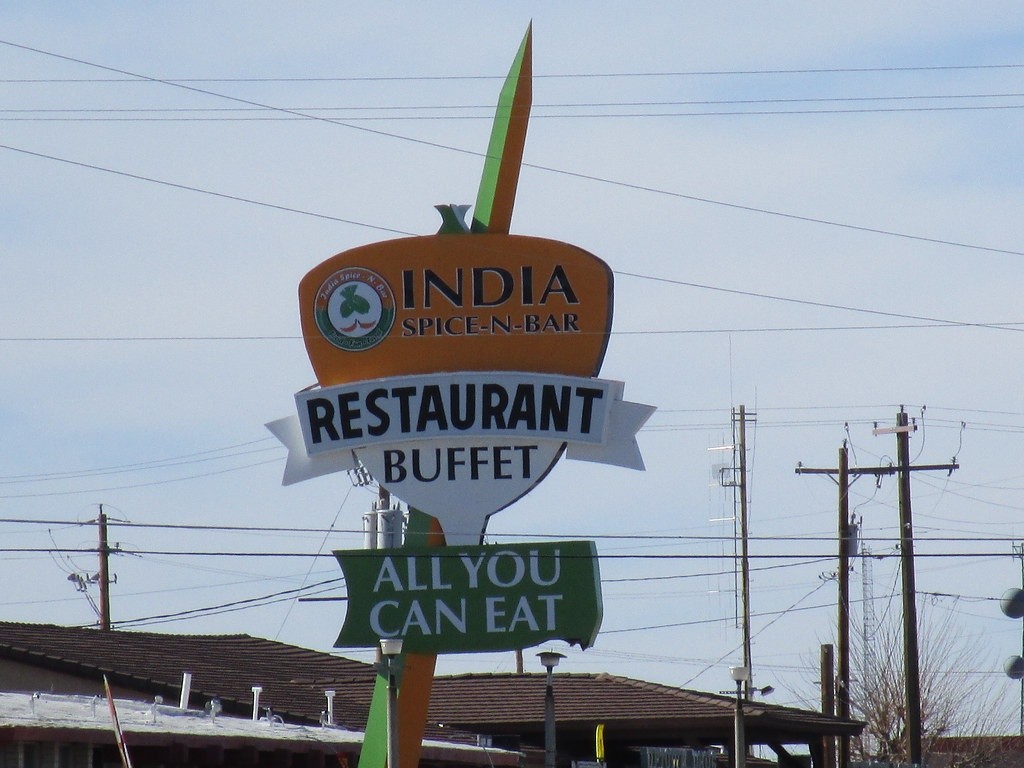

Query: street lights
745,687,775,700
731,667,748,768
537,651,568,767
379,637,405,768
